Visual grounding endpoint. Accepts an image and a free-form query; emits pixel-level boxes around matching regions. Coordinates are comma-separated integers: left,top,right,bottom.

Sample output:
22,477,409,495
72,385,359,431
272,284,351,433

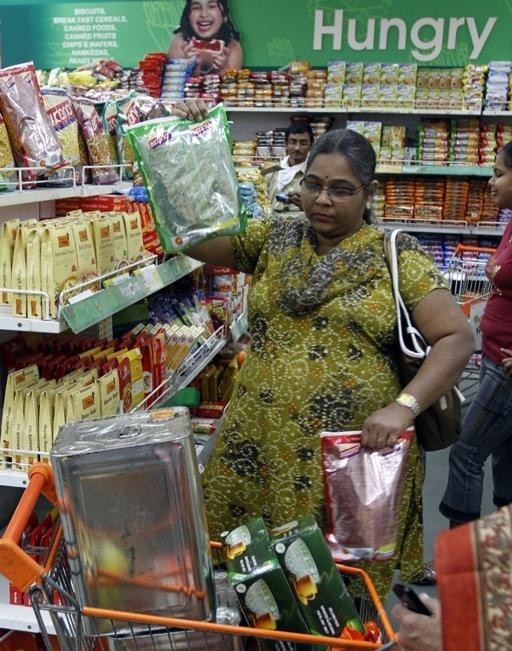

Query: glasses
300,176,368,203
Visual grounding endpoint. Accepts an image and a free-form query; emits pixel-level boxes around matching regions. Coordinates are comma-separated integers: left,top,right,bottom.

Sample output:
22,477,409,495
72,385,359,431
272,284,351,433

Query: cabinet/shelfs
223,98,512,284
0,95,253,650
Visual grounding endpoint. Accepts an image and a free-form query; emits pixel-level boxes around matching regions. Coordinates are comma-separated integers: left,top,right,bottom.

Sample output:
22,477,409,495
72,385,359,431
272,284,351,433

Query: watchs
393,391,422,416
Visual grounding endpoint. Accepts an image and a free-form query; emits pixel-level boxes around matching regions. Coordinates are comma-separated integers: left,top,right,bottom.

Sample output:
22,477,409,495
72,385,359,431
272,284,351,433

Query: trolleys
1,462,402,649
430,238,496,410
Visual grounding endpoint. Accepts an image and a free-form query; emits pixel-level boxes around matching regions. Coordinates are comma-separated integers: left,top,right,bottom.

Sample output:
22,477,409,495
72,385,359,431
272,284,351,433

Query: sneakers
411,563,437,587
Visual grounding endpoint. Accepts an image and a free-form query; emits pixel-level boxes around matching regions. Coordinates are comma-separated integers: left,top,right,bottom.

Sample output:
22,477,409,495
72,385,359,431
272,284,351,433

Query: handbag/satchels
393,303,462,453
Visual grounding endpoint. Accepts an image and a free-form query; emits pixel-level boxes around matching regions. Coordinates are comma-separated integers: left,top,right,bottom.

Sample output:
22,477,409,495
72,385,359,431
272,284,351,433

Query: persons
258,118,318,217
166,1,243,78
165,93,477,629
388,591,443,651
405,136,511,585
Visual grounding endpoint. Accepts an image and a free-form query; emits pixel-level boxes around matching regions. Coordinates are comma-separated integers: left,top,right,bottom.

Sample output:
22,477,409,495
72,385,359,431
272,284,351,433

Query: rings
376,435,386,442
388,435,398,442
